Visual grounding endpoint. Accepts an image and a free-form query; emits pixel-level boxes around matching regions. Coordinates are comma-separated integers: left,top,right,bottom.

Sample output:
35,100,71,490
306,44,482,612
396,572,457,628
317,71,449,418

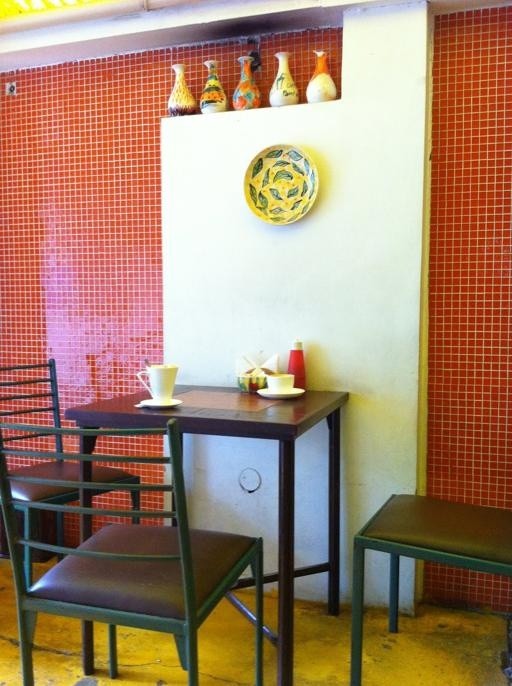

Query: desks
62,379,349,685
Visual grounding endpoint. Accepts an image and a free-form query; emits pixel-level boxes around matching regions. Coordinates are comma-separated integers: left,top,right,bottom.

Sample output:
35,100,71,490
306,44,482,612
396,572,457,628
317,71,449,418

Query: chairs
3,420,262,686
2,359,144,652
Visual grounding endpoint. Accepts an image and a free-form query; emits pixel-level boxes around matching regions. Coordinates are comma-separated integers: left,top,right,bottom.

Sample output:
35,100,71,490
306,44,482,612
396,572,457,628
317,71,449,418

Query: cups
263,373,294,393
136,364,179,403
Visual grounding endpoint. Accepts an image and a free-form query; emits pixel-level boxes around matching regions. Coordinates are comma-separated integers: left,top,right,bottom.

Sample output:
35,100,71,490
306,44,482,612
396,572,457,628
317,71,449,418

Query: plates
136,401,185,409
243,144,321,227
255,387,306,401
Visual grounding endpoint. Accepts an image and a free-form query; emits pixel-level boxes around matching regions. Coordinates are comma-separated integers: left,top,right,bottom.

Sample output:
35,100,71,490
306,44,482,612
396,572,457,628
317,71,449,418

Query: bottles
288,337,307,387
167,50,337,116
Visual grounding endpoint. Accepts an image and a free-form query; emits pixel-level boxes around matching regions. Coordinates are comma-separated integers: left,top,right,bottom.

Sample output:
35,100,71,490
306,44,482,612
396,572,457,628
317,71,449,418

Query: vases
166,46,336,119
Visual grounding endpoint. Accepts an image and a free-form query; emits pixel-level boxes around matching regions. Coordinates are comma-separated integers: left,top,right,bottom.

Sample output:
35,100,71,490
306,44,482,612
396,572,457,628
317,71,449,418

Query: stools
350,495,512,684
